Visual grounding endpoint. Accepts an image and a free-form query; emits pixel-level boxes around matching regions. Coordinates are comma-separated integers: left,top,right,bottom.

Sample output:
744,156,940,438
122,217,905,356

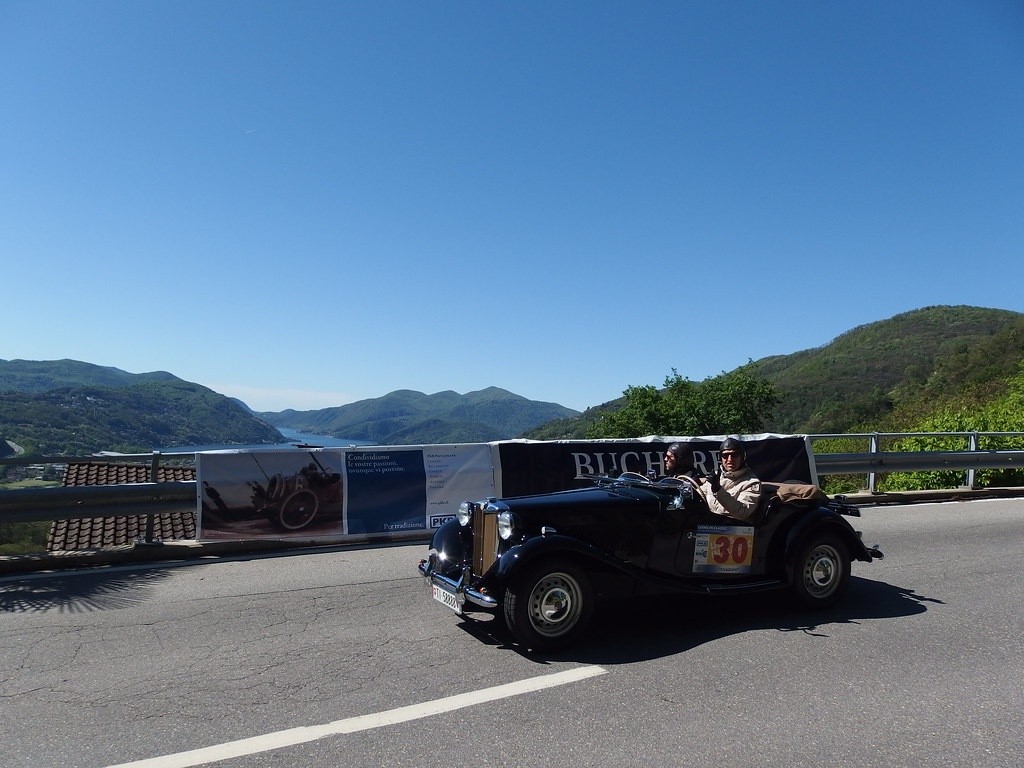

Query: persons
664,442,702,487
695,438,764,520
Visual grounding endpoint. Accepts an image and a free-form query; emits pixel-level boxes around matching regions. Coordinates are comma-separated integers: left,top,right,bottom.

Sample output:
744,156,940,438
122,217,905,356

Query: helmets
669,442,694,471
720,437,744,457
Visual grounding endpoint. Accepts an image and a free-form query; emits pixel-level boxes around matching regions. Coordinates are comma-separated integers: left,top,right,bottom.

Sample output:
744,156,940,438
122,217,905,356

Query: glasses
722,452,742,459
664,454,676,460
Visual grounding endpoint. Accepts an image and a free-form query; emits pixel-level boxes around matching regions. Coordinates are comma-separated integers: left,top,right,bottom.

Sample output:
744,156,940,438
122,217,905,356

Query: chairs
742,483,780,531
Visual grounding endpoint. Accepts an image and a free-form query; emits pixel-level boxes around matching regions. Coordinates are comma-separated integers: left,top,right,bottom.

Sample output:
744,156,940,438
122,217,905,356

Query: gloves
705,468,721,492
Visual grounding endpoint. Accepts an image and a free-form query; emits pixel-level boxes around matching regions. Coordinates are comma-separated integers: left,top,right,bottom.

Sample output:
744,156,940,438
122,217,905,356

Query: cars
249,459,345,531
419,470,884,650
